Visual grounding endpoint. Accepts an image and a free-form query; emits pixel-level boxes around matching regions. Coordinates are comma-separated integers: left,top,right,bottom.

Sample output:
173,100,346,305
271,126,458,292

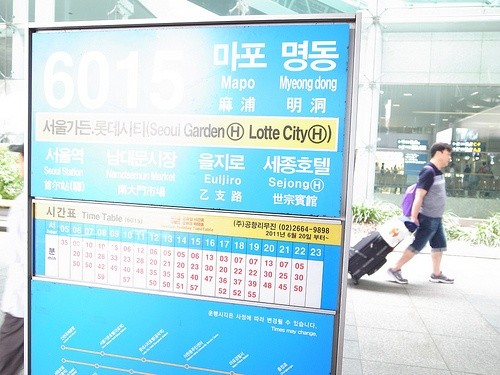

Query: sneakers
429,272,454,284
387,268,408,284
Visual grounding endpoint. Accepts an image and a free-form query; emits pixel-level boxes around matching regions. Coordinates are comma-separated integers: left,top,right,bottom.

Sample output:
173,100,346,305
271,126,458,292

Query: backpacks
402,166,434,217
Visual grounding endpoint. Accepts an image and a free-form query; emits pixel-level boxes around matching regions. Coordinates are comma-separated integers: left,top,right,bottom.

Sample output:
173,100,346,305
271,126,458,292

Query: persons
374,161,404,196
388,144,457,284
0,142,28,372
448,160,494,198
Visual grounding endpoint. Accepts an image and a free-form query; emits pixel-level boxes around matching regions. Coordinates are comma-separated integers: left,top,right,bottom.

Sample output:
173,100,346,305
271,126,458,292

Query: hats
9,145,24,156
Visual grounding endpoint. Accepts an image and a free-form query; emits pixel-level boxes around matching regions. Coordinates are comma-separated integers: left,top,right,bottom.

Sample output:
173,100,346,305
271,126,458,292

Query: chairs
445,177,464,196
477,179,500,198
375,173,410,194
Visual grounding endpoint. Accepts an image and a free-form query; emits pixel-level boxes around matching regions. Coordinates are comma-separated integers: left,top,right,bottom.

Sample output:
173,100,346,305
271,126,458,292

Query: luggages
348,224,419,286
